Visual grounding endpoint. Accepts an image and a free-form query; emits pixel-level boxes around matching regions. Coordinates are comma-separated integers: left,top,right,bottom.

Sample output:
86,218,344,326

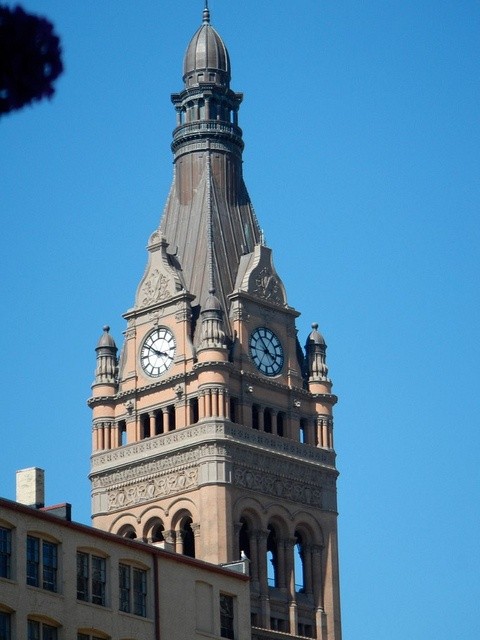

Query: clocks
246,325,285,377
138,324,177,380
